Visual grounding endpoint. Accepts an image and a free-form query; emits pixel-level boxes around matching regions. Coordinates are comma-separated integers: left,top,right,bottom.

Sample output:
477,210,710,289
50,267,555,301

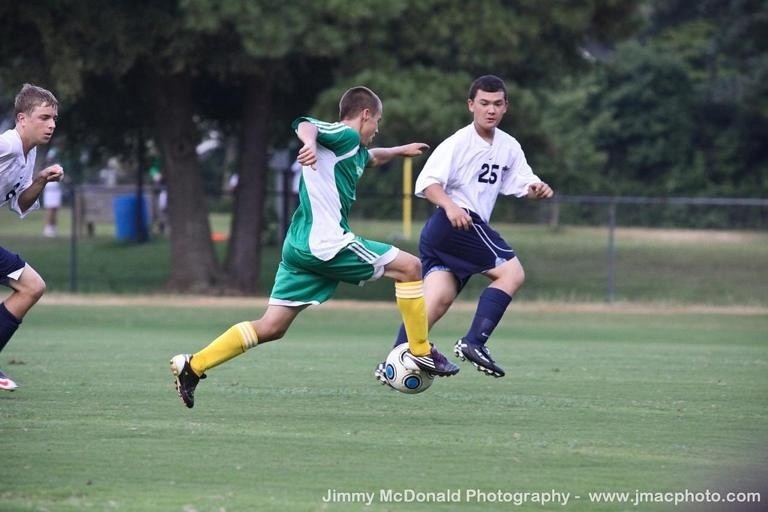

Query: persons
374,76,554,387
0,81,65,391
149,146,168,233
42,147,63,237
170,86,459,408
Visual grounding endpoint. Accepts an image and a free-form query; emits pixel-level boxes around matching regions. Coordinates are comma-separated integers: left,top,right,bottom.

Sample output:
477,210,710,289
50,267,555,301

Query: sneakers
400,342,460,377
453,337,506,377
375,361,391,386
170,353,199,407
0,370,18,391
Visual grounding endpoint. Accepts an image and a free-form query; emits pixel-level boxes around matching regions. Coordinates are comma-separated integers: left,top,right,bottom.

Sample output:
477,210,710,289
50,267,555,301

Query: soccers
386,341,434,393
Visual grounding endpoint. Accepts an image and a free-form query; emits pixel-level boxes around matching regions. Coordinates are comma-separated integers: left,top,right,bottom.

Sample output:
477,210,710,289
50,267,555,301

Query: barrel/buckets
112,192,151,242
